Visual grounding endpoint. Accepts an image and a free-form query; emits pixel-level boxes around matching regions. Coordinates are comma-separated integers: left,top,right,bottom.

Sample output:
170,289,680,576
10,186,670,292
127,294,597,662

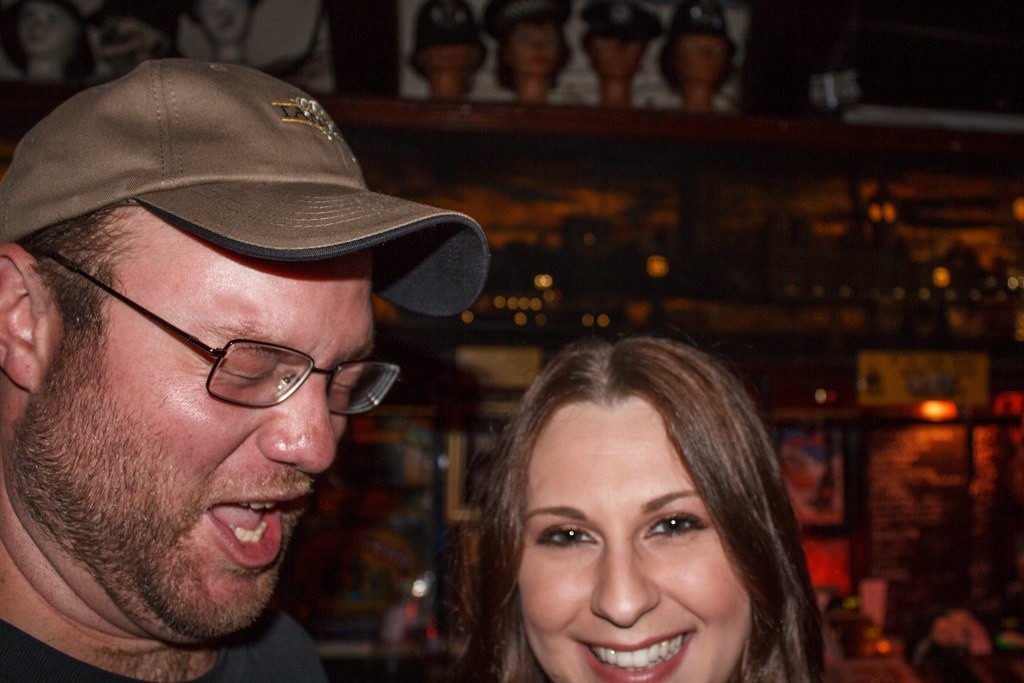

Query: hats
0,58,490,315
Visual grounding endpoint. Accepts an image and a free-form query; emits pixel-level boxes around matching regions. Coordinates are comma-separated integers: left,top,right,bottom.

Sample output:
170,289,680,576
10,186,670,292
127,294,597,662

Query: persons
1,56,491,682
580,0,663,109
658,0,737,112
409,0,486,99
439,333,826,683
480,0,574,103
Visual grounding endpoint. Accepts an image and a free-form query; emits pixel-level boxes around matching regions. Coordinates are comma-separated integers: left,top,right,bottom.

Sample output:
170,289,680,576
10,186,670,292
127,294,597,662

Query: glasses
16,236,399,415
